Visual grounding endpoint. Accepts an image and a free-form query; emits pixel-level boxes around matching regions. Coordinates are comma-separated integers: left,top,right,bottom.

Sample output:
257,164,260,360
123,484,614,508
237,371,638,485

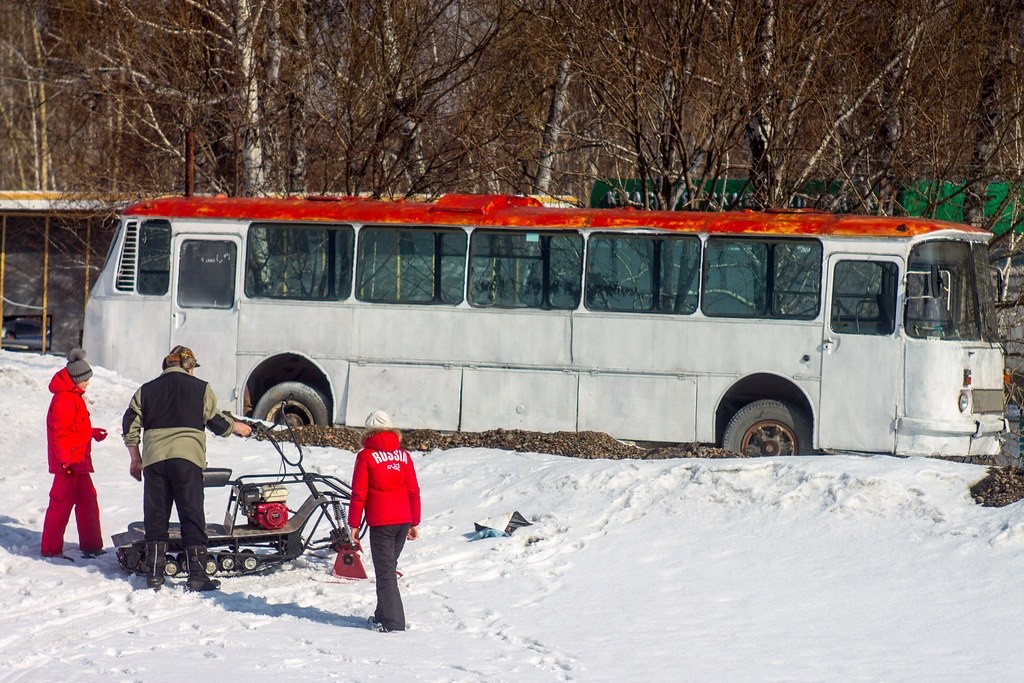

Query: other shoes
367,616,380,625
80,549,107,559
371,625,393,632
40,555,74,563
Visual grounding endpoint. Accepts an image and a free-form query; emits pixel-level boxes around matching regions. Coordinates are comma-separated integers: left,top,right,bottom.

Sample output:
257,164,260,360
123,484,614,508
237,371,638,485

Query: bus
80,192,1009,455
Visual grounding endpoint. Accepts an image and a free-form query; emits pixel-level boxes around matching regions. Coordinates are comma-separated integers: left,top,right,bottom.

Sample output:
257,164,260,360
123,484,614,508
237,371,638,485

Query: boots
183,545,221,592
146,540,168,591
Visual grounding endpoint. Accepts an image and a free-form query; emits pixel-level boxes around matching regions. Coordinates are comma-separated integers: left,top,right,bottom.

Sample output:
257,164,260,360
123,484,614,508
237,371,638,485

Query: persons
347,409,422,635
121,343,253,591
40,345,107,564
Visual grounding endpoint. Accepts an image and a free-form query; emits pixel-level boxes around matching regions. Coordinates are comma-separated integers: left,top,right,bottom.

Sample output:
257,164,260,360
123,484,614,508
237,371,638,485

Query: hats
162,345,200,371
365,410,393,431
66,348,94,383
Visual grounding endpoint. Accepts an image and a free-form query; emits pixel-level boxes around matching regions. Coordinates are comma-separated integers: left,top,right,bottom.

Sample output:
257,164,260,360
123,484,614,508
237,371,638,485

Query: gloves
92,428,107,441
62,462,75,478
